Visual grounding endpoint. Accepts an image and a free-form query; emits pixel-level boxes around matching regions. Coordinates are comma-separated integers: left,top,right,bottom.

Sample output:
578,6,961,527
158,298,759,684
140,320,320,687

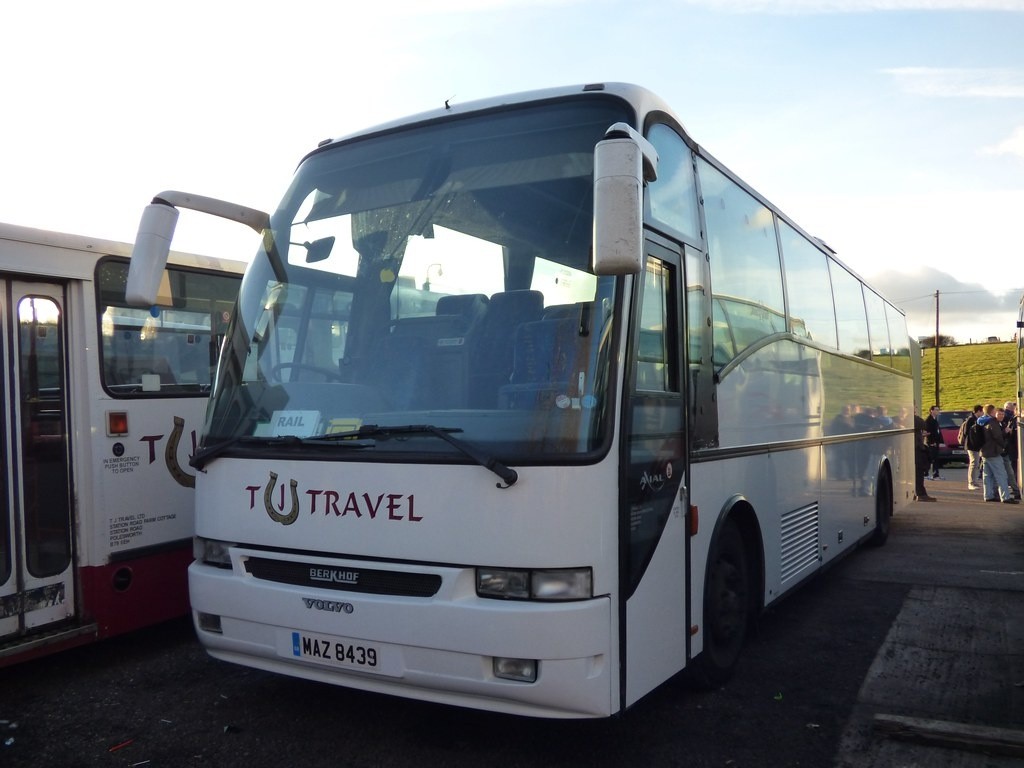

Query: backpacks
967,419,990,451
957,417,974,444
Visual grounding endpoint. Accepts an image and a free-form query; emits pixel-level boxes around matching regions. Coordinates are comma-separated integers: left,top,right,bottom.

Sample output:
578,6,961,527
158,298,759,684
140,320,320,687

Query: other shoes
1004,497,1019,504
933,475,945,481
968,484,981,490
1014,494,1021,499
917,495,936,502
913,495,916,501
924,475,933,480
985,497,1000,502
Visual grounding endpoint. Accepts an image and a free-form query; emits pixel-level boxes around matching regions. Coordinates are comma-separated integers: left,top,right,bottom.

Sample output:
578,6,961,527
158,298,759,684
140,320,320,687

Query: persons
914,400,937,502
1004,403,1019,495
963,405,983,490
993,408,1022,500
977,405,1020,504
830,403,909,495
923,406,947,481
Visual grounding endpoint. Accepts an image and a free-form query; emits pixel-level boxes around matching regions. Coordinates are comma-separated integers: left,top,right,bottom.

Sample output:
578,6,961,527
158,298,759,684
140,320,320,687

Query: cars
933,409,971,467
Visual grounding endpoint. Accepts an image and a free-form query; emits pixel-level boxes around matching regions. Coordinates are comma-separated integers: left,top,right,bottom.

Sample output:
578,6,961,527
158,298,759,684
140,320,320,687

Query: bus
0,221,453,666
124,80,915,723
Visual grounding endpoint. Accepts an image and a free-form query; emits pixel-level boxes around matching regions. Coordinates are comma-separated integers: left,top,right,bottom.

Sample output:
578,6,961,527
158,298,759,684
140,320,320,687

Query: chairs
384,289,594,414
